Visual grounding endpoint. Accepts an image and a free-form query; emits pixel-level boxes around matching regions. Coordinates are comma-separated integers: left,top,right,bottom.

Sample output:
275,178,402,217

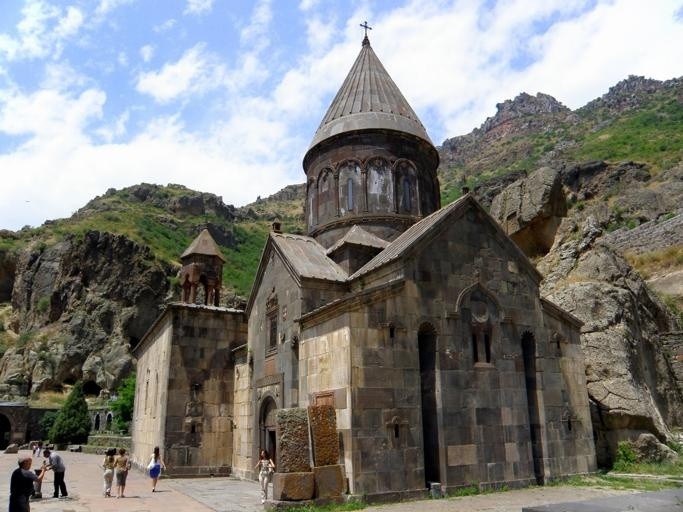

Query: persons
8,439,68,512
102,446,167,497
254,449,275,505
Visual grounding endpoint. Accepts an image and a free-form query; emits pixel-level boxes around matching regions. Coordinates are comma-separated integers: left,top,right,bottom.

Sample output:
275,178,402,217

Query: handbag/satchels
147,458,156,469
268,463,274,472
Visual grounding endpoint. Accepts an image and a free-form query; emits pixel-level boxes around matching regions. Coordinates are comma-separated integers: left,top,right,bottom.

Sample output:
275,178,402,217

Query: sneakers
51,494,68,498
